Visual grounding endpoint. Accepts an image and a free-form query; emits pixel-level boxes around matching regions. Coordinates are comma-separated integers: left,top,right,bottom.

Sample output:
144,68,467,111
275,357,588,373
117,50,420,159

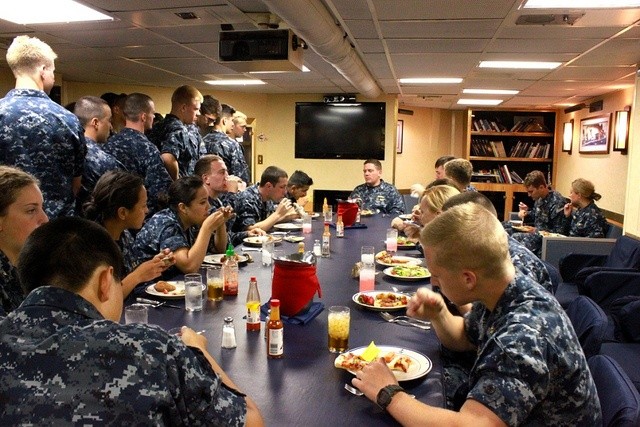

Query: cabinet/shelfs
470,184,509,224
462,107,561,186
509,187,554,219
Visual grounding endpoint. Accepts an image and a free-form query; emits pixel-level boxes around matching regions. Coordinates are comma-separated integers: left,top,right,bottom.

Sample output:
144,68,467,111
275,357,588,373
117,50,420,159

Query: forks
384,311,431,325
343,383,416,400
131,301,167,309
379,312,431,330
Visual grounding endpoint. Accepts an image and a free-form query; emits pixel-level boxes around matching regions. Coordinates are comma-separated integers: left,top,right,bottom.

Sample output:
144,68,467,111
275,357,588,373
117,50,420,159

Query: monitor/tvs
296,94,386,160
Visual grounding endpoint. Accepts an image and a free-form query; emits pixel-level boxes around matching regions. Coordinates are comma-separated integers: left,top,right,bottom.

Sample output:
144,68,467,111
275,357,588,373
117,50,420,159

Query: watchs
373,379,402,410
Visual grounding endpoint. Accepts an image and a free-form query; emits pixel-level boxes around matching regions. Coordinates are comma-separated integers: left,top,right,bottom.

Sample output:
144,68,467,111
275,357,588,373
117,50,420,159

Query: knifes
241,248,284,252
137,297,182,309
271,232,299,235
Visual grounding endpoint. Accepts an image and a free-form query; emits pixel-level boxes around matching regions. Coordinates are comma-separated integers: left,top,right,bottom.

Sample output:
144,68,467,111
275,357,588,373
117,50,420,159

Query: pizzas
391,264,427,277
376,250,409,263
335,351,410,373
396,236,412,244
374,292,408,308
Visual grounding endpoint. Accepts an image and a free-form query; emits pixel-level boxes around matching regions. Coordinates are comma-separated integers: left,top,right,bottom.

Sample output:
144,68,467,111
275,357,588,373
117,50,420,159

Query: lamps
614,105,631,155
561,119,573,155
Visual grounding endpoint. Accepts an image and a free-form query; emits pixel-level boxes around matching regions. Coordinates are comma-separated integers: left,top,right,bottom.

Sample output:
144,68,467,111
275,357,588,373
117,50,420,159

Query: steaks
155,280,176,292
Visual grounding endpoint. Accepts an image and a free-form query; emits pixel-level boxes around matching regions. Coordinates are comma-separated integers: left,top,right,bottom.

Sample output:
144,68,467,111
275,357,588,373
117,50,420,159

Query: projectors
218,29,311,71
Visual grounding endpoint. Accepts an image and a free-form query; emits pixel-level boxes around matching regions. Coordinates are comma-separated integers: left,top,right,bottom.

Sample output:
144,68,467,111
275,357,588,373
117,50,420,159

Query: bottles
322,198,328,217
354,207,361,223
298,242,305,253
220,317,238,350
313,239,321,256
222,243,238,296
246,277,261,333
265,299,285,358
335,213,345,237
321,222,331,258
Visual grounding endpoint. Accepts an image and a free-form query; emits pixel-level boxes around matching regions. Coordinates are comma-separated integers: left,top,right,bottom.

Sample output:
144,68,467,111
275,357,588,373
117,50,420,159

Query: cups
124,304,148,327
361,246,375,268
324,205,333,222
184,272,203,312
303,216,312,234
206,267,224,302
327,304,351,353
168,327,181,335
261,235,275,266
235,182,247,191
386,228,398,251
359,268,375,292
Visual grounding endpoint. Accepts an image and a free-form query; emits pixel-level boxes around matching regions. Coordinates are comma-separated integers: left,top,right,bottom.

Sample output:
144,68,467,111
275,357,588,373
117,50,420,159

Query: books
480,165,509,182
470,136,551,159
546,164,552,183
509,169,524,183
472,115,546,132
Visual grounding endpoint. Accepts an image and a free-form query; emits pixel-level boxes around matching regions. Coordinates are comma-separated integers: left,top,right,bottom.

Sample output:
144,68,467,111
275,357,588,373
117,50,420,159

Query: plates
352,290,412,312
376,255,423,266
243,235,282,245
399,213,416,219
203,253,249,266
401,221,420,228
383,267,432,281
511,226,530,232
144,280,206,300
384,238,418,246
359,212,376,216
539,231,568,238
302,213,320,218
273,222,303,230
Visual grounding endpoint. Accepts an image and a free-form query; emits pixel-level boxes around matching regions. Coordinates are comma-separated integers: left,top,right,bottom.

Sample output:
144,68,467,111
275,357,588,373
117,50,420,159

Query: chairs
603,341,640,388
590,354,640,427
567,296,615,357
559,235,637,297
583,273,640,343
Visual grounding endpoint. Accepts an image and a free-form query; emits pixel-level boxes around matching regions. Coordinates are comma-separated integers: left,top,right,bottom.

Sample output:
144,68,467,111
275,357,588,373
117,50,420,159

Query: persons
73,93,130,201
404,184,466,241
231,108,248,138
1,214,264,426
147,82,204,181
426,178,466,190
287,170,313,208
350,200,606,425
131,176,236,277
203,103,249,185
444,158,479,191
0,32,88,218
434,153,457,180
1,164,51,314
234,164,298,230
83,169,177,297
193,154,270,241
101,91,127,133
102,93,177,203
512,176,610,250
348,159,408,215
501,170,563,234
441,190,553,291
199,95,222,134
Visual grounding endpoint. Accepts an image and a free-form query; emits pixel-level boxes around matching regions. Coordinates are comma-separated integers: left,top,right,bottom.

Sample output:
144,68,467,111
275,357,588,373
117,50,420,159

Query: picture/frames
578,114,611,155
396,118,404,154
